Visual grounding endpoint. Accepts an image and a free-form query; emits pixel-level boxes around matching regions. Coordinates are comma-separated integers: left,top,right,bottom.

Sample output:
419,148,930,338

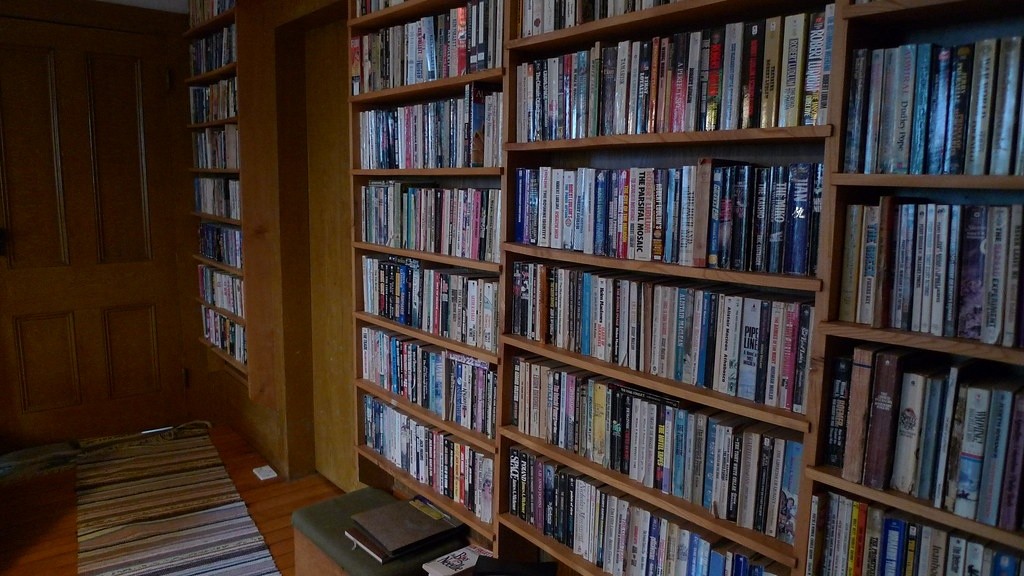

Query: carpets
73,421,281,576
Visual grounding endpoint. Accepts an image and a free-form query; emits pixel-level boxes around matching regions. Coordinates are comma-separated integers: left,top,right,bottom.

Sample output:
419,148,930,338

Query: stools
290,487,471,576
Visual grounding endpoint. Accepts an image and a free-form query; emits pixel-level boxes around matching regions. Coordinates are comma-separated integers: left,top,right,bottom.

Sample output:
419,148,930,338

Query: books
351,0,506,530
804,37,1024,576
185,1,247,365
497,0,836,576
345,497,467,566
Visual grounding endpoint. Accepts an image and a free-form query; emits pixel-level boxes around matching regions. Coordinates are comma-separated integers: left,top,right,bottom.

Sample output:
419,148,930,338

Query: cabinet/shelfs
181,6,248,380
346,0,1024,576
